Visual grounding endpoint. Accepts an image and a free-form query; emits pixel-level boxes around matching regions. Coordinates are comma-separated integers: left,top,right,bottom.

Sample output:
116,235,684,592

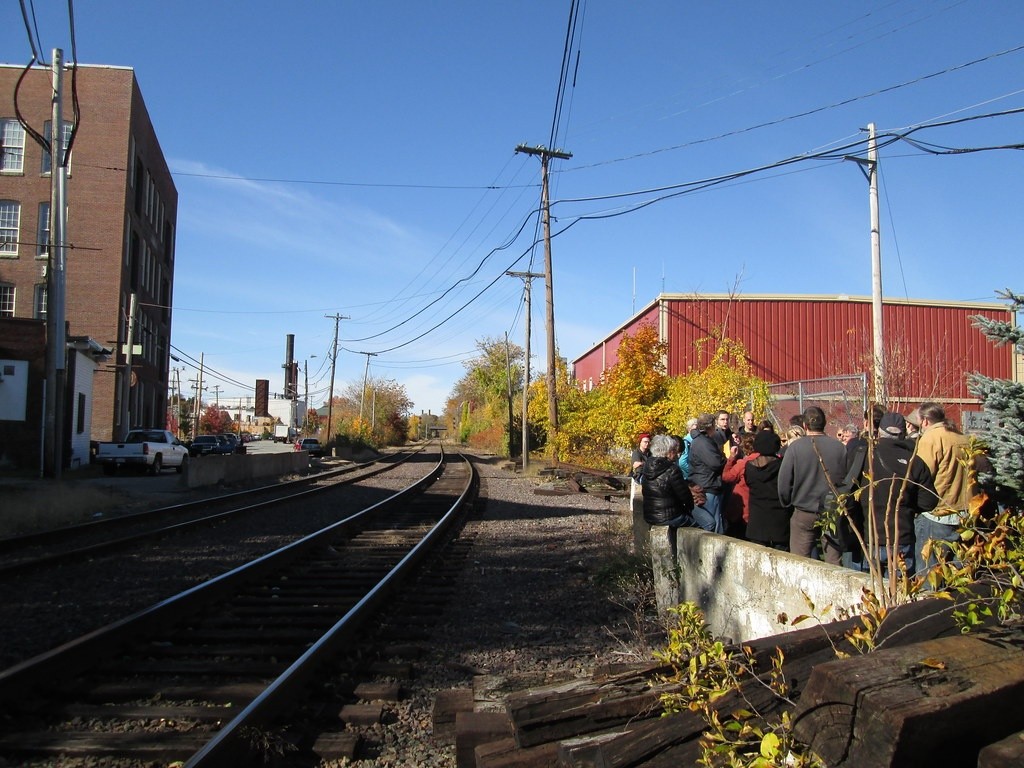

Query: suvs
217,432,242,456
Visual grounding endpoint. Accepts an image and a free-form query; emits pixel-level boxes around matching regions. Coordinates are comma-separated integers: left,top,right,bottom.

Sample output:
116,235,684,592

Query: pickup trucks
98,429,190,476
301,439,322,456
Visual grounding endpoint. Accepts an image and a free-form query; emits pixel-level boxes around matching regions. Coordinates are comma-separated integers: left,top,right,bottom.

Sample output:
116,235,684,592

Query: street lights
173,366,186,440
289,383,308,438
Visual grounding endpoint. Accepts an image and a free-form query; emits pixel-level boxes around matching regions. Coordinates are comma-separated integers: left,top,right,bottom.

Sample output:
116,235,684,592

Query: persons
631,402,1000,591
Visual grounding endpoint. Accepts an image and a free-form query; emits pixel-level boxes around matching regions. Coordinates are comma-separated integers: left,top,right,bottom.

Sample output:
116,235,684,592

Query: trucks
274,424,288,443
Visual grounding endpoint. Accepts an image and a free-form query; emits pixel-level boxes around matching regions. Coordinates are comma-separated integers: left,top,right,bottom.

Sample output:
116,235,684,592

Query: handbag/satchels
821,487,863,552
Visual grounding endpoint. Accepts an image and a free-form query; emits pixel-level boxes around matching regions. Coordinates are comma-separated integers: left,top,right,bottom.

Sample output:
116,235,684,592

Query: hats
903,409,918,427
753,430,781,456
639,433,652,441
879,413,906,436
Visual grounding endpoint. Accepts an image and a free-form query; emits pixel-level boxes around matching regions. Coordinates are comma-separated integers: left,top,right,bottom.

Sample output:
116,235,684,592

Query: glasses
838,433,843,437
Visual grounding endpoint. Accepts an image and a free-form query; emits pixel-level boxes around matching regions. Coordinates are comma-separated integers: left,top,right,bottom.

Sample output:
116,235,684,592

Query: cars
189,435,222,458
241,433,262,443
